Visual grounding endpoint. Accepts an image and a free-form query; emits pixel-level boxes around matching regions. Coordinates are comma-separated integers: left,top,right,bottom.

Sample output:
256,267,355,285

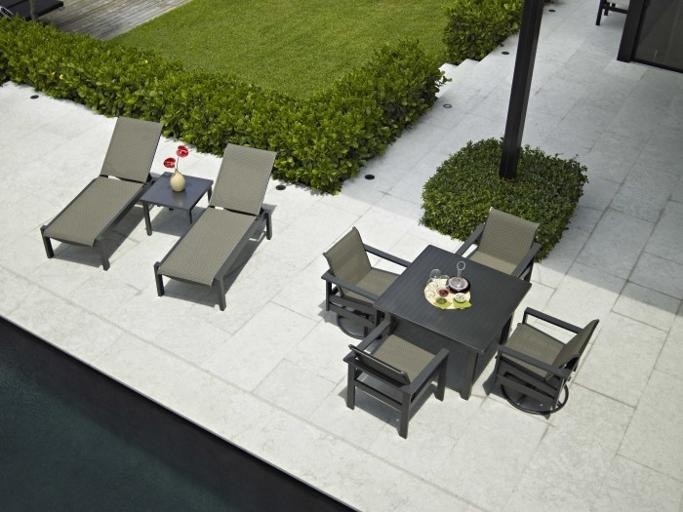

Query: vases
168,170,186,195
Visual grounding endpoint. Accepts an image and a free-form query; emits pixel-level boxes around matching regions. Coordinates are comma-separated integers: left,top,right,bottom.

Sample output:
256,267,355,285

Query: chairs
455,207,542,282
153,142,277,313
492,307,600,411
40,113,165,272
322,226,413,338
342,316,451,439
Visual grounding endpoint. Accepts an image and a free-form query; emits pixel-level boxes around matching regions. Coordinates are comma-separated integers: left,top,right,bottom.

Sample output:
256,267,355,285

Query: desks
373,244,532,400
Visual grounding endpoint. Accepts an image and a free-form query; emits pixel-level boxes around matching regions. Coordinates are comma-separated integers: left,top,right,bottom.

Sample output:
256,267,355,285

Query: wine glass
426,260,471,305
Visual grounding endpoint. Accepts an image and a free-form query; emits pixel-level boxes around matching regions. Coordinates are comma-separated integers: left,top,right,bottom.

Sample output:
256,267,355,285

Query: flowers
162,144,189,171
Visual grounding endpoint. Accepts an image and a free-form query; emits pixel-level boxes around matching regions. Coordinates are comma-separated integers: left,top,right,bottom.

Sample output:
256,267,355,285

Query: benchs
40,113,165,272
153,142,277,313
